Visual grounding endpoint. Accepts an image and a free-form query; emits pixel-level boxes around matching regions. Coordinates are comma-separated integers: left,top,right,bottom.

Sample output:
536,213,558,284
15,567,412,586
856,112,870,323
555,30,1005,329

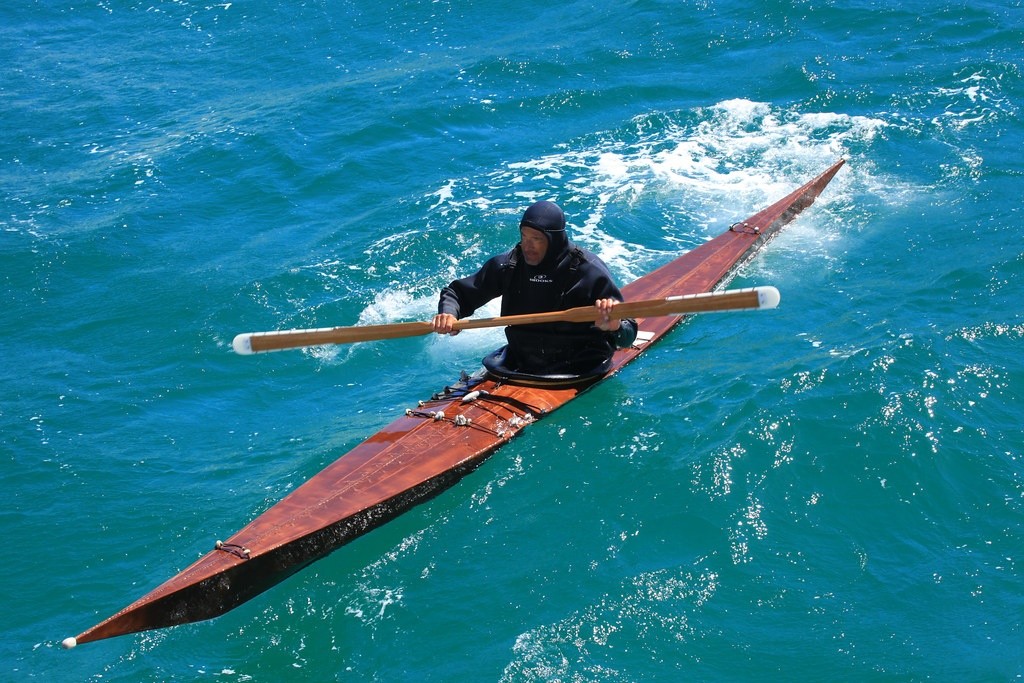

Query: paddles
232,287,782,356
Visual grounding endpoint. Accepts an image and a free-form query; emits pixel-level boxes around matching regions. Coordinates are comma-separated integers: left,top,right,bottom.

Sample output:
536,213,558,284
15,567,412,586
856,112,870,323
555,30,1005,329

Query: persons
430,200,638,375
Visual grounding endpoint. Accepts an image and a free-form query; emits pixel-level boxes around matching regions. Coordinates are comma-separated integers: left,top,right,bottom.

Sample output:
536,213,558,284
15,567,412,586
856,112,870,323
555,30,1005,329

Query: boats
60,152,854,653
